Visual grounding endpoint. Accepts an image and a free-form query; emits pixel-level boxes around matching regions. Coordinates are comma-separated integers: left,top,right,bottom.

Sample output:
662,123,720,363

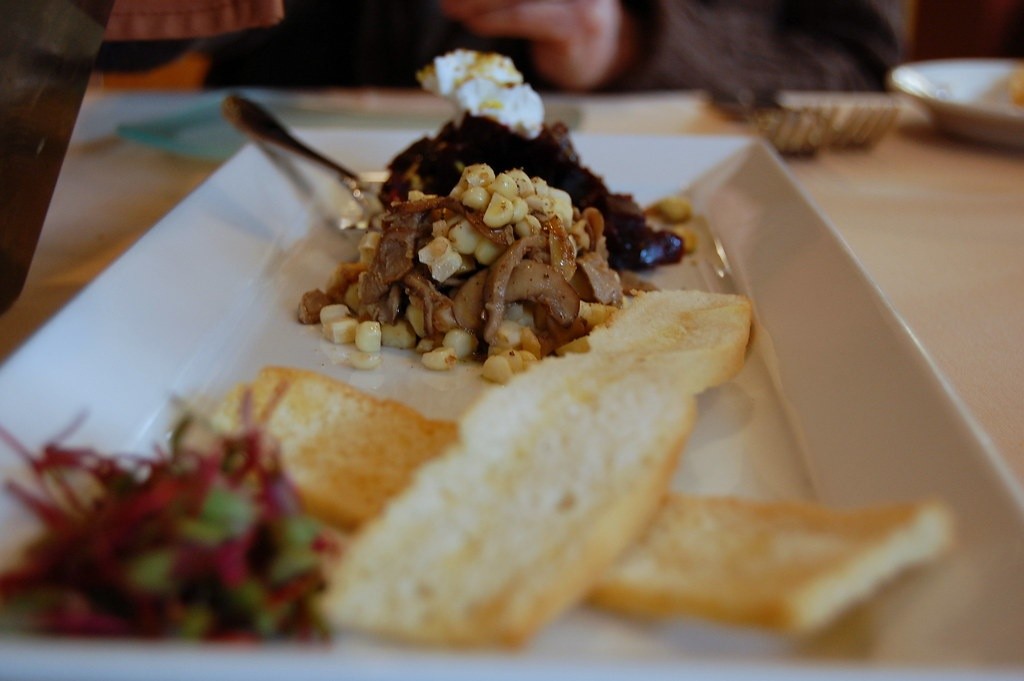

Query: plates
0,133,1023,679
892,58,1023,143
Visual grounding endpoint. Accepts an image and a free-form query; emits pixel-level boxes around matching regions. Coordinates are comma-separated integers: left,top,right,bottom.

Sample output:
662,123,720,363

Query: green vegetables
0,383,340,646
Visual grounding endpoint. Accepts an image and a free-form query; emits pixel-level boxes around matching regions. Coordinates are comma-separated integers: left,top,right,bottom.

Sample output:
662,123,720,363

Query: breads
309,292,754,654
188,365,951,631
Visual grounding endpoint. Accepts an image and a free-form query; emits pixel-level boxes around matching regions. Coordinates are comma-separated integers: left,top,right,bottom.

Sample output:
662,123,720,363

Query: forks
832,104,901,148
732,76,838,158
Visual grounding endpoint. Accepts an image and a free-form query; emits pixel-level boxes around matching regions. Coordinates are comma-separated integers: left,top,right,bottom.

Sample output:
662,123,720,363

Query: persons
207,0,891,117
